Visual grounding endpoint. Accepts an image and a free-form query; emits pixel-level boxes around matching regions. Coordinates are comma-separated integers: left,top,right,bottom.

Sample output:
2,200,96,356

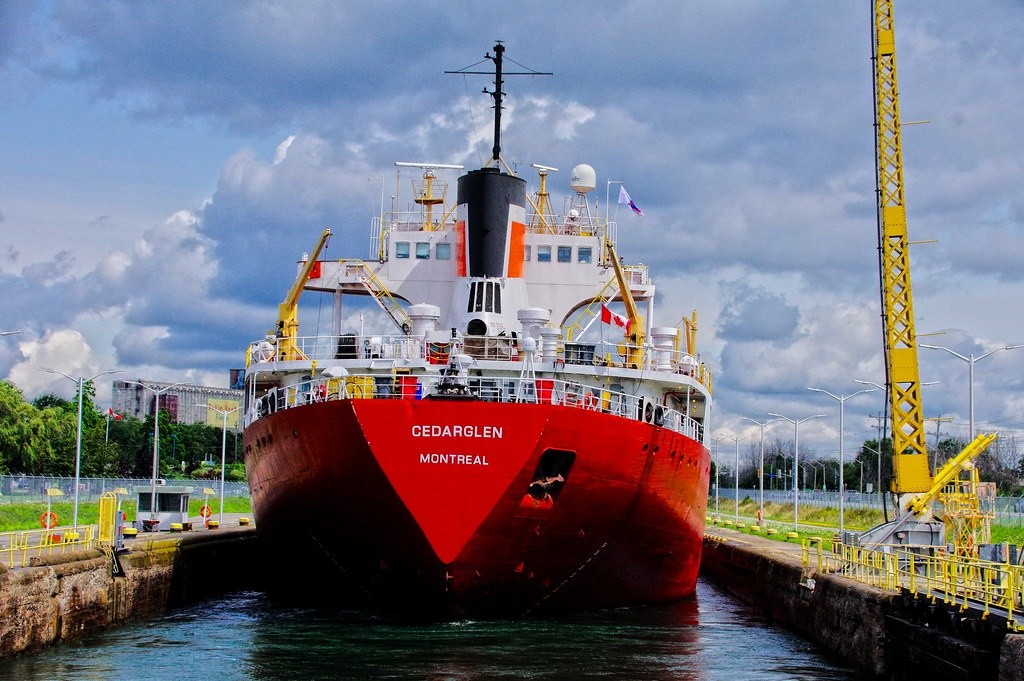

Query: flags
599,303,633,335
617,184,644,217
108,407,122,420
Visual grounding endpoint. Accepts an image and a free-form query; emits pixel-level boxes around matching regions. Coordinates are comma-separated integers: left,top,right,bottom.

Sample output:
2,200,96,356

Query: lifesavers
200,506,212,517
585,391,596,408
40,512,58,528
316,384,327,403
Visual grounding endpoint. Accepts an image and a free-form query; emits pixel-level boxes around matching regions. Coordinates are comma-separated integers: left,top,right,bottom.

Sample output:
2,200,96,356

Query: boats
238,48,708,610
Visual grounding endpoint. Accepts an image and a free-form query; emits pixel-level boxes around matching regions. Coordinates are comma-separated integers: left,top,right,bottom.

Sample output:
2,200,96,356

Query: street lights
38,367,123,532
708,434,755,520
802,453,826,491
739,416,793,520
808,385,880,533
0,330,21,336
853,377,938,390
121,378,193,522
803,461,816,491
195,400,246,525
857,443,881,502
919,342,1024,546
768,411,827,528
